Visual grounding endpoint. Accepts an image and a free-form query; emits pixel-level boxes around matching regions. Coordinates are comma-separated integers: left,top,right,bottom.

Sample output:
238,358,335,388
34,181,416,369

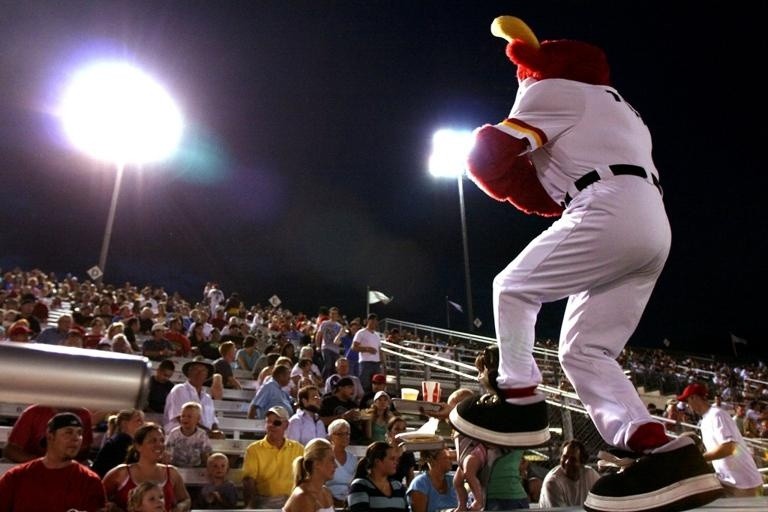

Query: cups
420,380,439,403
399,387,419,402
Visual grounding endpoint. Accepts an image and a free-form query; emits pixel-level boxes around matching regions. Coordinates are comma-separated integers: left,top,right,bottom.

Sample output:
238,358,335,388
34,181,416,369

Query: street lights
54,60,183,283
426,123,481,334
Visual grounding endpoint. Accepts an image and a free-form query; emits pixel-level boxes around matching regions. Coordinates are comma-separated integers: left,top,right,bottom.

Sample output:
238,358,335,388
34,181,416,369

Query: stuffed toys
446,14,727,510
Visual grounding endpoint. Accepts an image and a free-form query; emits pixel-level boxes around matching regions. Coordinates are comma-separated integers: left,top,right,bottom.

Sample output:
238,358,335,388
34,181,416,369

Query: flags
369,290,394,305
448,301,464,313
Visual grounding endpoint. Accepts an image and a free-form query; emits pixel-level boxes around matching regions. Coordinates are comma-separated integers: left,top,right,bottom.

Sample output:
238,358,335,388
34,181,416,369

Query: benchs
139,358,256,411
384,350,482,392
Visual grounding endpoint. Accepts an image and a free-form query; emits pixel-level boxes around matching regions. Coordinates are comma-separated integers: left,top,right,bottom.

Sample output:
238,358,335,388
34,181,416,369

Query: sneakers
448,342,552,450
585,446,725,512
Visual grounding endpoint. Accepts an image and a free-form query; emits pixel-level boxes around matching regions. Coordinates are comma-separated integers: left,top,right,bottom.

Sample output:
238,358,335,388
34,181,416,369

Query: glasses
306,394,323,400
266,420,284,426
333,432,351,437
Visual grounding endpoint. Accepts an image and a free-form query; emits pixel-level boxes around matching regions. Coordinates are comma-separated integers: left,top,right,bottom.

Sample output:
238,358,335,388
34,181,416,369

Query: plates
394,432,436,440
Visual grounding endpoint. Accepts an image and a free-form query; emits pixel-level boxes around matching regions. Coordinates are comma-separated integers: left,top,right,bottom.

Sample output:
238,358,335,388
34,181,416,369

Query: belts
561,164,664,209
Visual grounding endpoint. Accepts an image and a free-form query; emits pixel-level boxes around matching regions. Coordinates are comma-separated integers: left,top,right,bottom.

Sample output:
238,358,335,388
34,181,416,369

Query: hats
676,383,706,400
38,412,83,449
299,346,314,362
152,323,169,331
10,326,33,336
182,355,213,379
372,373,386,387
265,405,289,421
373,391,389,402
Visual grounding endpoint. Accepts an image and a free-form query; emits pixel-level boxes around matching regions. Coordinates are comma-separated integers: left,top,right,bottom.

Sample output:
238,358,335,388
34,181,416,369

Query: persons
711,401,768,437
146,360,174,412
276,357,295,395
202,453,238,509
283,438,338,511
257,352,280,389
292,346,322,382
2,265,141,356
365,391,400,446
444,388,488,510
746,438,768,490
0,413,106,511
334,320,360,376
383,415,407,454
324,358,364,404
324,420,359,511
351,314,387,391
320,379,364,443
4,404,95,462
212,341,244,391
140,282,212,362
427,344,531,512
387,328,427,378
315,307,343,375
235,335,261,371
427,335,467,362
283,386,327,444
301,314,315,346
538,437,601,510
678,384,764,498
517,451,542,501
361,374,394,407
536,340,577,394
620,342,768,400
241,406,305,510
249,365,292,417
313,304,330,334
291,359,322,386
212,283,244,341
258,306,301,357
101,422,193,512
165,402,211,468
678,401,700,435
127,478,165,512
405,446,468,512
298,378,313,386
92,407,145,477
346,441,411,511
245,303,258,335
647,399,678,434
163,363,226,439
